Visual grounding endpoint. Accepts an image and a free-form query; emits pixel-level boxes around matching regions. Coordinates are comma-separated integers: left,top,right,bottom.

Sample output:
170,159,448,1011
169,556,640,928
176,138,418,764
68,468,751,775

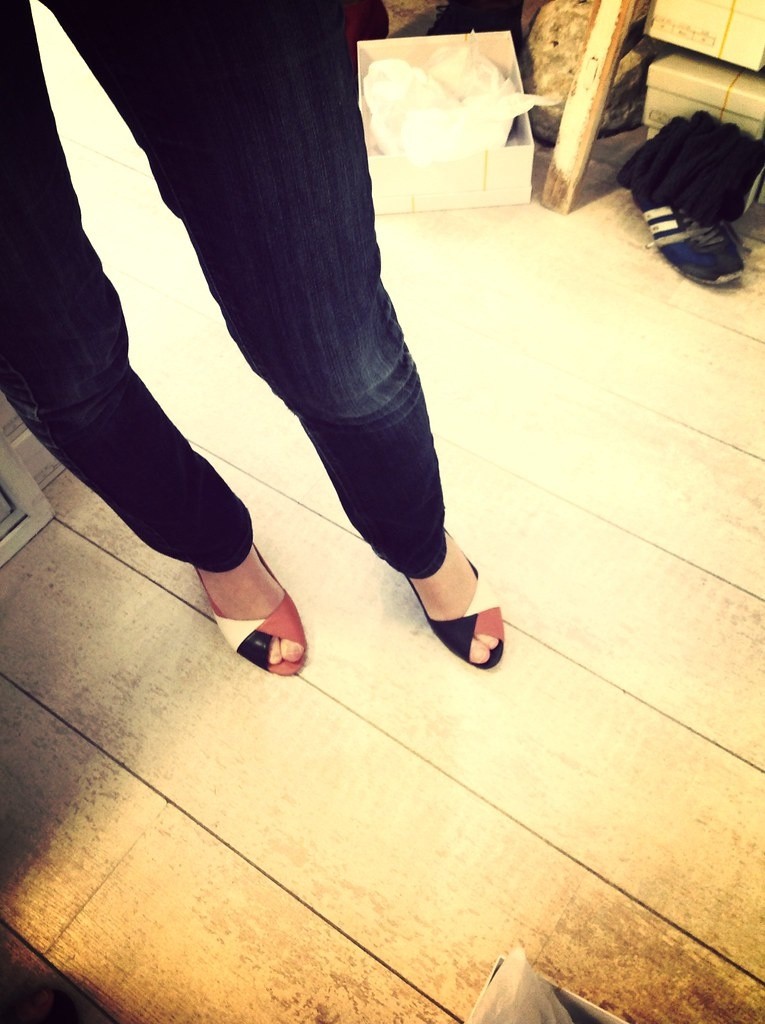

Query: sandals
193,544,308,676
405,527,505,670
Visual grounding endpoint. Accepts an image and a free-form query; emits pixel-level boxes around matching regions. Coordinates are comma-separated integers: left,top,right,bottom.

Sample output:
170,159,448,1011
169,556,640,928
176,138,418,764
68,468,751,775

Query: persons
0,0,506,675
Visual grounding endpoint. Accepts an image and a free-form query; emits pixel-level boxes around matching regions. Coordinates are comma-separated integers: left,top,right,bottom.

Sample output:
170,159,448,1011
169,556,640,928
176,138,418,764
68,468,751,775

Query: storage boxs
643,0,765,72
356,30,536,215
642,45,765,139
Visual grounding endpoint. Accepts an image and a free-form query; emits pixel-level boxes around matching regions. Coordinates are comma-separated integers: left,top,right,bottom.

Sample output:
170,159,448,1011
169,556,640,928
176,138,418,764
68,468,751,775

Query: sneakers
632,186,745,285
